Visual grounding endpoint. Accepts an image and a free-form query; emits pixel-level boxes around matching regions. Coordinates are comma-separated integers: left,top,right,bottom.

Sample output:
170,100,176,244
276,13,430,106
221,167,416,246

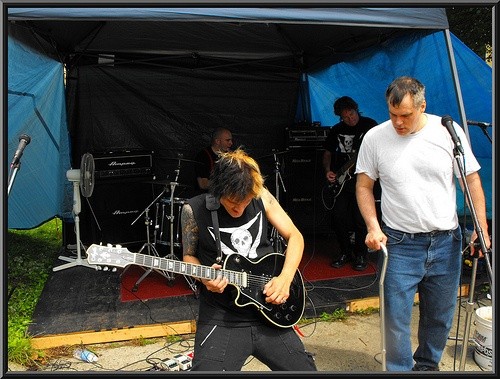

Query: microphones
11,133,30,168
466,120,492,127
441,114,465,154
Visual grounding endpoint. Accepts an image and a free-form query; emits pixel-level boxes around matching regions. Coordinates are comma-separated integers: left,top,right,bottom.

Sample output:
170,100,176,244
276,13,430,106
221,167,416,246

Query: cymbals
155,154,197,162
139,180,190,187
258,149,291,157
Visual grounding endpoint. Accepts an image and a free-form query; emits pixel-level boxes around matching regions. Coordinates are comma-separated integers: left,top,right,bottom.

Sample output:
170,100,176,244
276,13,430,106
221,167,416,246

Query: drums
153,198,191,248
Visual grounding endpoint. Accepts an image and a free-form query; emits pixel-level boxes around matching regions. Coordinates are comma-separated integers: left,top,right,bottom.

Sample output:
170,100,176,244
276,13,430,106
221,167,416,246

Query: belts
414,229,458,237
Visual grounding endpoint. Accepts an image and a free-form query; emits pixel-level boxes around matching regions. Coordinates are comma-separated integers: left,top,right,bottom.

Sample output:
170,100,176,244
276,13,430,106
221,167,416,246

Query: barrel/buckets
474,305,492,370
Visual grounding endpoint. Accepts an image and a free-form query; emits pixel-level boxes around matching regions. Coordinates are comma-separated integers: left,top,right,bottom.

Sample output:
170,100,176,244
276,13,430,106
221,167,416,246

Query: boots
351,232,368,270
331,229,352,268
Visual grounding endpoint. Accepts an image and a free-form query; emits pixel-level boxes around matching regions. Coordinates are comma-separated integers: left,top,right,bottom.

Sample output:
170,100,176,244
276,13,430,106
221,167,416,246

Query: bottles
73,347,98,363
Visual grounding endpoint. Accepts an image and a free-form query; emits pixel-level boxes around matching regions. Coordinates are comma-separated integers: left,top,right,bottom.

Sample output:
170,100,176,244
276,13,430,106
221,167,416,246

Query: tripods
266,148,288,255
117,160,199,299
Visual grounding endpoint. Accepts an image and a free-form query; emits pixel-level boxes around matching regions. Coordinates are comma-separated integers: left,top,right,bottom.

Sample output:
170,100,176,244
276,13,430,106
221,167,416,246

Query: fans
53,153,101,271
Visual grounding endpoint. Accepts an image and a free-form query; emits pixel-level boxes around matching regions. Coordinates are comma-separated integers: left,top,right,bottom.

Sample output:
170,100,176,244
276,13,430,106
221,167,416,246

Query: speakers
278,151,338,236
88,170,162,248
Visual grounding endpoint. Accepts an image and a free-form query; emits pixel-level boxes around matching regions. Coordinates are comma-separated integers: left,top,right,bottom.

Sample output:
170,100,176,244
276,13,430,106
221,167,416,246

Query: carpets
120,244,380,303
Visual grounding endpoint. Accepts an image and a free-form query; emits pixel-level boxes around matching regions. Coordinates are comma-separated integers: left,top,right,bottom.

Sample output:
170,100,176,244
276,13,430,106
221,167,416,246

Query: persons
353,75,490,372
322,96,379,271
181,148,318,372
193,126,233,196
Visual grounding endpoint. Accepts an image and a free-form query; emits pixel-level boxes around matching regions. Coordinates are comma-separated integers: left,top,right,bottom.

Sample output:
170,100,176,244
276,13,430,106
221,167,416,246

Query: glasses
340,109,355,122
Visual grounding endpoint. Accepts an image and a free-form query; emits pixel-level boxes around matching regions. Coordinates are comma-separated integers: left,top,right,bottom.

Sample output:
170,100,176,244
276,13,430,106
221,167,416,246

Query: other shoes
412,364,437,372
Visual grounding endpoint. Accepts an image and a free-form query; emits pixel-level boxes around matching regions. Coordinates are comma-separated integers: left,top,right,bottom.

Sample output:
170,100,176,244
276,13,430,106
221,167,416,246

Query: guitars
320,154,357,198
86,241,306,328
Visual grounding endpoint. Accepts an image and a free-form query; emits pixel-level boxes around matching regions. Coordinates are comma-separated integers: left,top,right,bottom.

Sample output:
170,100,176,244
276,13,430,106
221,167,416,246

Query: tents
7,5,492,231
